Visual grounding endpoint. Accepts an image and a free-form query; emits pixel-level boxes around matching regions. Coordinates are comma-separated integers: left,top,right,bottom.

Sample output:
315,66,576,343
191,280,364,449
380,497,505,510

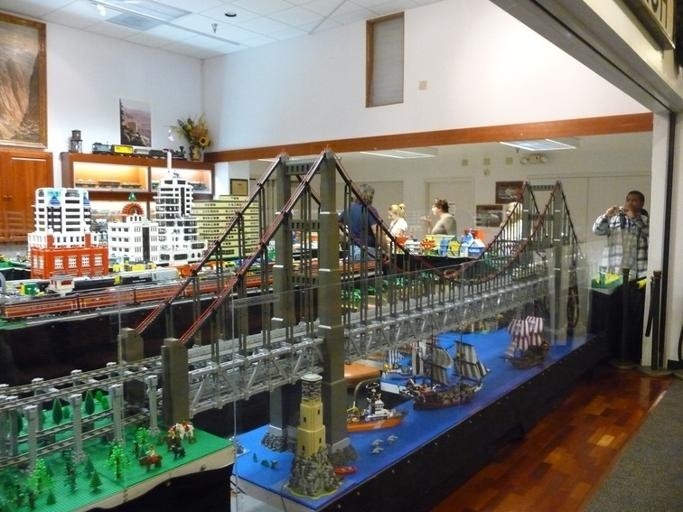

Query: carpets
573,375,682,511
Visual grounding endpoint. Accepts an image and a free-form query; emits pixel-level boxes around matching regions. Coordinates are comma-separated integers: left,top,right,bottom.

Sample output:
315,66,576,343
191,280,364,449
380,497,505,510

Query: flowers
169,115,214,148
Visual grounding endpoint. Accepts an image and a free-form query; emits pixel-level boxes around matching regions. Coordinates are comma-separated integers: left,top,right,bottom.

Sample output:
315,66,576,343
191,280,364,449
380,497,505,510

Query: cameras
618,206,625,215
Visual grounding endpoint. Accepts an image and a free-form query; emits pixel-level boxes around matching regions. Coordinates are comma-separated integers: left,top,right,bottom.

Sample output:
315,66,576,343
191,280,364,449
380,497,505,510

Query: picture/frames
0,12,48,150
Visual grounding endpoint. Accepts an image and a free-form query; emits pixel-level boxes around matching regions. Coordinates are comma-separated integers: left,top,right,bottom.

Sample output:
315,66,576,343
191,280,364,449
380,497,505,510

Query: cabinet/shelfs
0,145,214,244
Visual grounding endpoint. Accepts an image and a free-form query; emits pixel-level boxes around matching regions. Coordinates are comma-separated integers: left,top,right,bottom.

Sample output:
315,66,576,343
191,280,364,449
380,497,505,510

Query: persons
495,202,522,257
383,203,408,256
592,191,648,278
336,183,381,265
419,198,456,235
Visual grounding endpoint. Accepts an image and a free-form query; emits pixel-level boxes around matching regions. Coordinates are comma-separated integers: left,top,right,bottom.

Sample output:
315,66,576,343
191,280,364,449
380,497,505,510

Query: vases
190,145,204,162
